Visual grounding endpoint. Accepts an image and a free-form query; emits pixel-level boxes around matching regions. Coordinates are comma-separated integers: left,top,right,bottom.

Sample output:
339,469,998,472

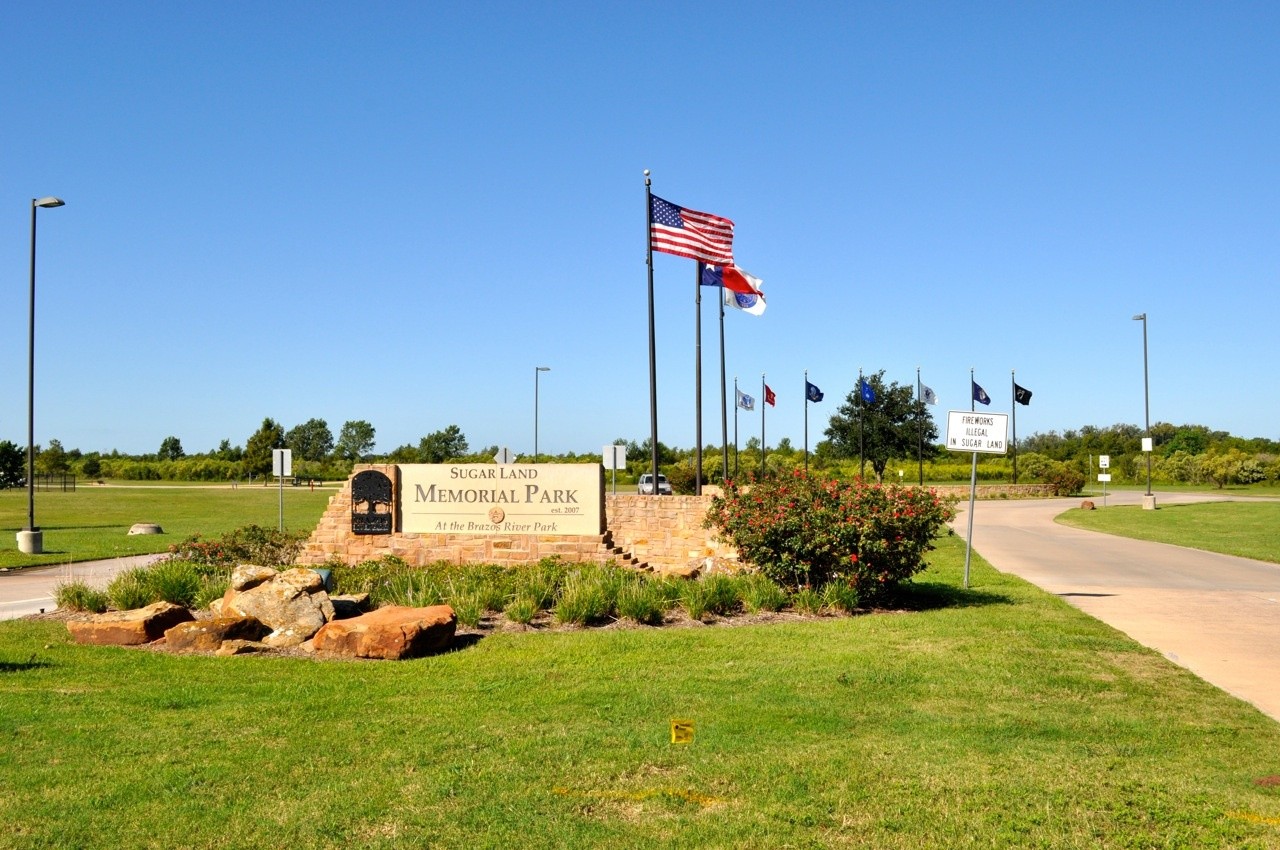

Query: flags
973,381,991,405
806,381,824,402
861,380,876,403
1014,383,1033,405
764,384,777,407
649,192,735,268
698,261,767,315
738,389,755,410
920,383,938,405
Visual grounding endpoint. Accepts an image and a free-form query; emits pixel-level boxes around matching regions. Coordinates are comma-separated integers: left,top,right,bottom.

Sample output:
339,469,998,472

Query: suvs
637,473,673,496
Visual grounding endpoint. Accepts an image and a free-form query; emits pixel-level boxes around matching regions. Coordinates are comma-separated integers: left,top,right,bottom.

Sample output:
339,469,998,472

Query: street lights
1131,312,1154,497
535,366,551,462
19,197,66,531
